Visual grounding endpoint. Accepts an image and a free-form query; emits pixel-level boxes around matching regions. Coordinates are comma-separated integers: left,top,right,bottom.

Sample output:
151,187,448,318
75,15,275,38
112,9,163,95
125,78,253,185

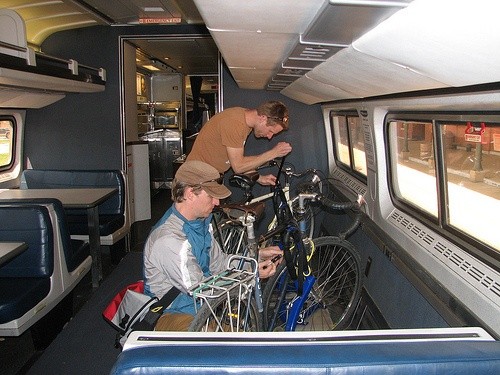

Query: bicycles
213,161,322,255
187,174,366,332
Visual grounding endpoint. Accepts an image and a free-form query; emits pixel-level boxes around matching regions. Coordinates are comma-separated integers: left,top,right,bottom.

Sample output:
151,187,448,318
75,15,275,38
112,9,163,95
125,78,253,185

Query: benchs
0,169,500,374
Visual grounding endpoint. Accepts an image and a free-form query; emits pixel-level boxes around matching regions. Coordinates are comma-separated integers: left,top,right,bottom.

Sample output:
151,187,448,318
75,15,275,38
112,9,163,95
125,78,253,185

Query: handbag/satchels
102,280,164,336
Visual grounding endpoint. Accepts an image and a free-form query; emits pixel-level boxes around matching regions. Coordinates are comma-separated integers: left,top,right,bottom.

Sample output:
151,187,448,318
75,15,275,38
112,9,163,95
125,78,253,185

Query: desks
0,241,28,265
0,188,119,288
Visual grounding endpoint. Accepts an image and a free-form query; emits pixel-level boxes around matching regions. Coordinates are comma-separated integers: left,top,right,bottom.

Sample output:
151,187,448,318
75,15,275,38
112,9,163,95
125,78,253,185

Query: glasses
267,115,289,123
202,173,224,184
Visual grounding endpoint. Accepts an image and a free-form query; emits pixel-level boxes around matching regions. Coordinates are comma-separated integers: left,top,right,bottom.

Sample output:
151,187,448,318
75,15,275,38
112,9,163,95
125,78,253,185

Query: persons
142,160,284,332
187,100,293,186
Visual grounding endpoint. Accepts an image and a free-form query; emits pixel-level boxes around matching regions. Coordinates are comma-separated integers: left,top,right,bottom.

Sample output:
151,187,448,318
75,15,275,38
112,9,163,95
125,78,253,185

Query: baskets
311,177,365,236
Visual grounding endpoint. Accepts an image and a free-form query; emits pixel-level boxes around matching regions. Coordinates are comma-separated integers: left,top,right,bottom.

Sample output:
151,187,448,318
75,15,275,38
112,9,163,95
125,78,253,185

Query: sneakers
224,303,252,329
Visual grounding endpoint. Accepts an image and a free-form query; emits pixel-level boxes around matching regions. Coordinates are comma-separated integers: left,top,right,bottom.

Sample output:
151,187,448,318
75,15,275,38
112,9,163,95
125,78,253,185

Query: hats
175,161,232,199
